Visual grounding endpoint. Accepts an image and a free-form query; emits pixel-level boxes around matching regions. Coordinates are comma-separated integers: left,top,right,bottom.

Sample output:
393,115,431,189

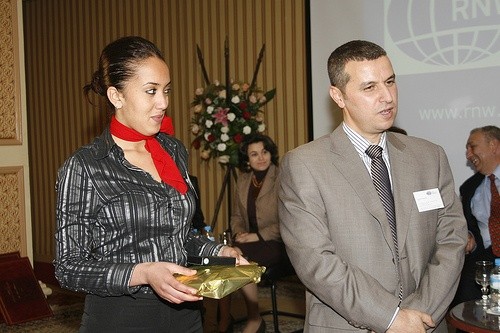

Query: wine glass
475,261,493,306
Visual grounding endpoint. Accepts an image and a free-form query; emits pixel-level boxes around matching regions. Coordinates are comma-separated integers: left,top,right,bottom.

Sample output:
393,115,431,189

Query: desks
445,299,500,333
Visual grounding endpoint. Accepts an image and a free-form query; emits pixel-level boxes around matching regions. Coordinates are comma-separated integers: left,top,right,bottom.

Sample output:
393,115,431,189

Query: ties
365,145,405,301
488,174,500,257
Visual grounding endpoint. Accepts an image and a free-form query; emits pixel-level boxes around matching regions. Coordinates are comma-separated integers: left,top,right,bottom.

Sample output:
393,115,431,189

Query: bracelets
214,243,228,256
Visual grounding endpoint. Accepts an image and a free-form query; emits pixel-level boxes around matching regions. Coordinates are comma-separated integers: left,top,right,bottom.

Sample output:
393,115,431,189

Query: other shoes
256,320,266,333
212,315,234,333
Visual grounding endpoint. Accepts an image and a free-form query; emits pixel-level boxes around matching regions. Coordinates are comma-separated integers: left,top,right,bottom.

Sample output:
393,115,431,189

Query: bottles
490,258,500,313
203,226,215,242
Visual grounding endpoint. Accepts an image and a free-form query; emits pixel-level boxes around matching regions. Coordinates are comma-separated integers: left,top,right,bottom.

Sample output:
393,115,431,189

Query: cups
218,232,233,248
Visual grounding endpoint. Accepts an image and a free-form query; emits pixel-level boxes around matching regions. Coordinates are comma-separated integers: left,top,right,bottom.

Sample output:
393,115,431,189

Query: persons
459,126,500,296
277,41,468,333
217,133,290,333
53,36,251,333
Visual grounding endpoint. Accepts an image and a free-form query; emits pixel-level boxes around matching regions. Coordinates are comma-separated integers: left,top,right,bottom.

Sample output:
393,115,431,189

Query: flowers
188,78,267,170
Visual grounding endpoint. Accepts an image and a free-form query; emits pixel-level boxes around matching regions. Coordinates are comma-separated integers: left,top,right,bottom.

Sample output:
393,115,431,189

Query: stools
231,262,307,333
